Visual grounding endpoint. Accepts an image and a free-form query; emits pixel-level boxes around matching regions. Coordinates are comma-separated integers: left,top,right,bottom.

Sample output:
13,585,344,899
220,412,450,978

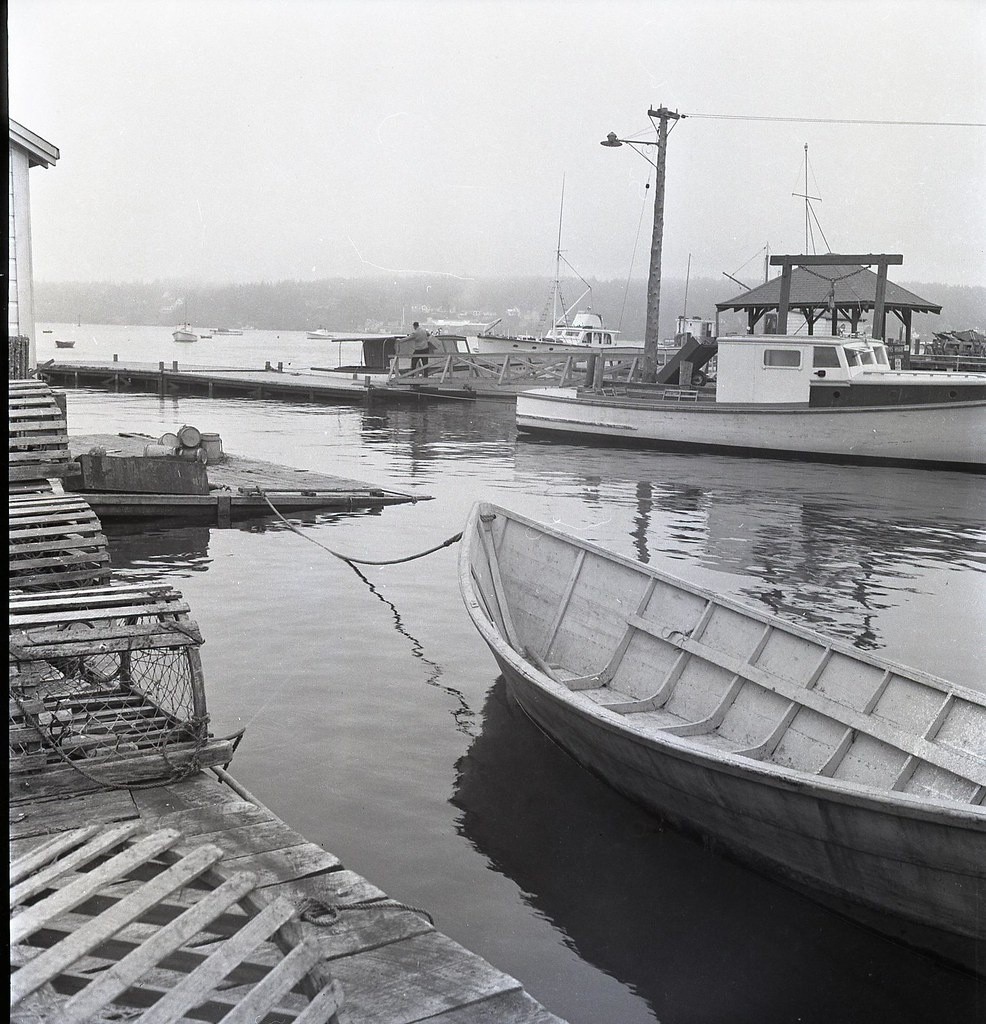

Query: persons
396,322,430,379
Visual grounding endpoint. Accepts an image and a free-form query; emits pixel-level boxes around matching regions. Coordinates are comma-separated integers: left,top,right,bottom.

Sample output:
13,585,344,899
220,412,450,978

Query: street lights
601,108,680,383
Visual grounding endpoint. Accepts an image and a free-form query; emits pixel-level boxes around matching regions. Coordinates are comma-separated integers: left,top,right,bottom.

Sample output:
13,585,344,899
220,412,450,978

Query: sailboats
172,290,198,342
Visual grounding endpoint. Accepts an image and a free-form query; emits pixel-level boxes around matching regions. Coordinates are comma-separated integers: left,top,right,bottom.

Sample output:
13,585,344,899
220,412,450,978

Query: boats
306,324,338,340
210,329,243,336
477,306,682,372
457,500,985,979
56,341,75,348
515,334,986,470
310,334,501,383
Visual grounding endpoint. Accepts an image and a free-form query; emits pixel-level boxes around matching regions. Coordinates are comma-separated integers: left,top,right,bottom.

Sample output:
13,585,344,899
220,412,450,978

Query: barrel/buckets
145,424,222,464
